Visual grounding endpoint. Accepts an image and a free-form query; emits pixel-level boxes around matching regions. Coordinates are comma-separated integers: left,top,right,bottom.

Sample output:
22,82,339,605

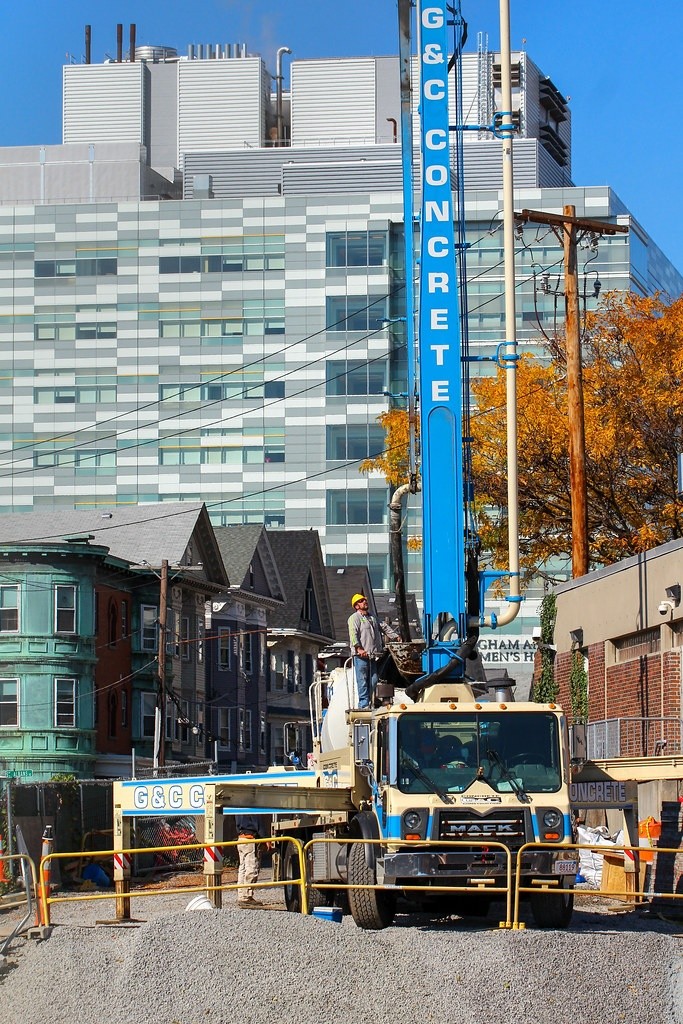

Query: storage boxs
311,906,342,924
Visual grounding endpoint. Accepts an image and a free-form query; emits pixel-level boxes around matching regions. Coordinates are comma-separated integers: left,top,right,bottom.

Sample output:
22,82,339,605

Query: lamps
658,600,674,615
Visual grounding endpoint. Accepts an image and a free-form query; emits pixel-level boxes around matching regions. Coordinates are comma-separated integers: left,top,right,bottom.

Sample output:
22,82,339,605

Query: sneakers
240,898,264,907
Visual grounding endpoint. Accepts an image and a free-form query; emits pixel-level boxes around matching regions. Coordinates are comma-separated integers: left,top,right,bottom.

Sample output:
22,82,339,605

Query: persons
348,593,402,710
234,816,271,905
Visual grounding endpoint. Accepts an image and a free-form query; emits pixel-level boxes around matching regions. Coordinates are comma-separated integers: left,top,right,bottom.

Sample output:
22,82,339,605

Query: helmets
351,594,368,608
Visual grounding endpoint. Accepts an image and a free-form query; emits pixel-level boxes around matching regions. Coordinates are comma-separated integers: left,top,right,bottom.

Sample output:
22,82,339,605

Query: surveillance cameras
659,605,668,615
532,627,542,641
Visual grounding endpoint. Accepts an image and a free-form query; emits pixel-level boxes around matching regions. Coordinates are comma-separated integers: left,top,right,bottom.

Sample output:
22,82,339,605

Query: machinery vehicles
271,0,580,932
